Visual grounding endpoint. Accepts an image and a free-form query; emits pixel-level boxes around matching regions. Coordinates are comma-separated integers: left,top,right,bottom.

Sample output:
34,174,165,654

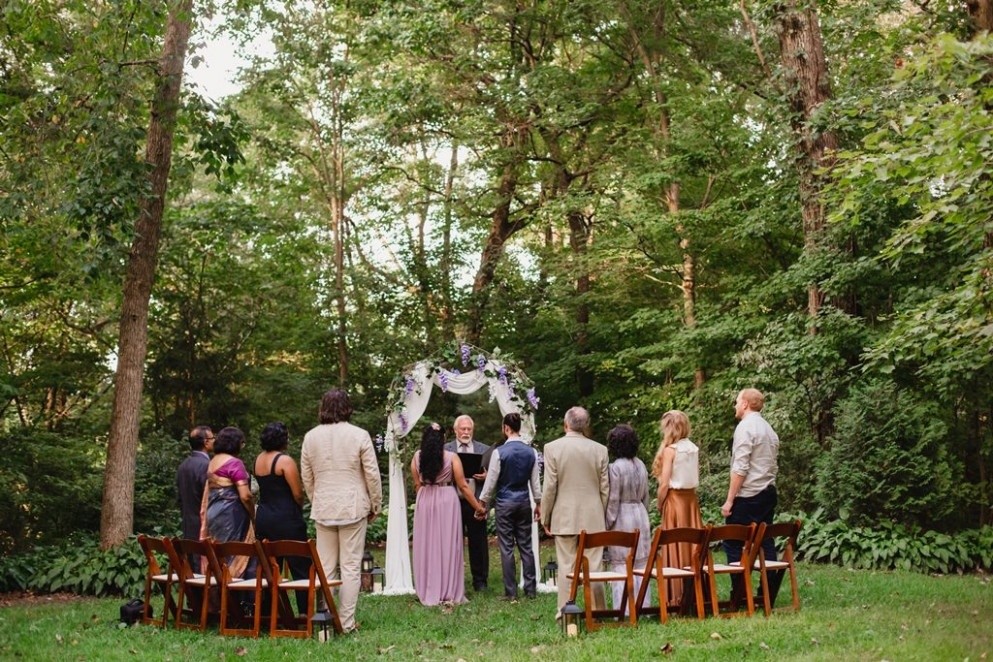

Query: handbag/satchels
120,598,154,627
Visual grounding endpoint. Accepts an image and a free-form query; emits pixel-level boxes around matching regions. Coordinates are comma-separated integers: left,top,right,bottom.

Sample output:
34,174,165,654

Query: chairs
563,519,803,632
136,533,345,638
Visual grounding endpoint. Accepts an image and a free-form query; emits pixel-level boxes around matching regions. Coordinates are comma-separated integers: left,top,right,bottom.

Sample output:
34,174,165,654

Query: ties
459,443,470,483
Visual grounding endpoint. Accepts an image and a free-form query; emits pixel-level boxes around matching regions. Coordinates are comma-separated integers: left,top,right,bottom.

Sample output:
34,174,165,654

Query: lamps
309,600,339,630
542,555,558,586
560,601,583,639
362,552,384,593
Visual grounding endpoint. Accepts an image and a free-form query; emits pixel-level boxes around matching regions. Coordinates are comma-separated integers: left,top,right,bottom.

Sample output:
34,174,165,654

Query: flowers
374,340,540,476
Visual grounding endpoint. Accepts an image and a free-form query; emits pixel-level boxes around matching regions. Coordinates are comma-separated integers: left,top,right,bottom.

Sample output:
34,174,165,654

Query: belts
420,481,453,486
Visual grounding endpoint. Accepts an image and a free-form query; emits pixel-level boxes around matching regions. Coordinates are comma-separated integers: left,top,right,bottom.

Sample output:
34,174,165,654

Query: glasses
203,436,215,440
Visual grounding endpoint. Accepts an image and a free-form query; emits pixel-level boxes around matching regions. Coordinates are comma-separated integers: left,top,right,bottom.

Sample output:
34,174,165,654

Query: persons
651,410,701,611
411,423,485,605
205,427,258,616
176,426,214,610
604,425,650,619
441,415,493,593
721,388,780,610
253,421,310,617
299,391,383,634
541,405,610,624
474,412,542,600
